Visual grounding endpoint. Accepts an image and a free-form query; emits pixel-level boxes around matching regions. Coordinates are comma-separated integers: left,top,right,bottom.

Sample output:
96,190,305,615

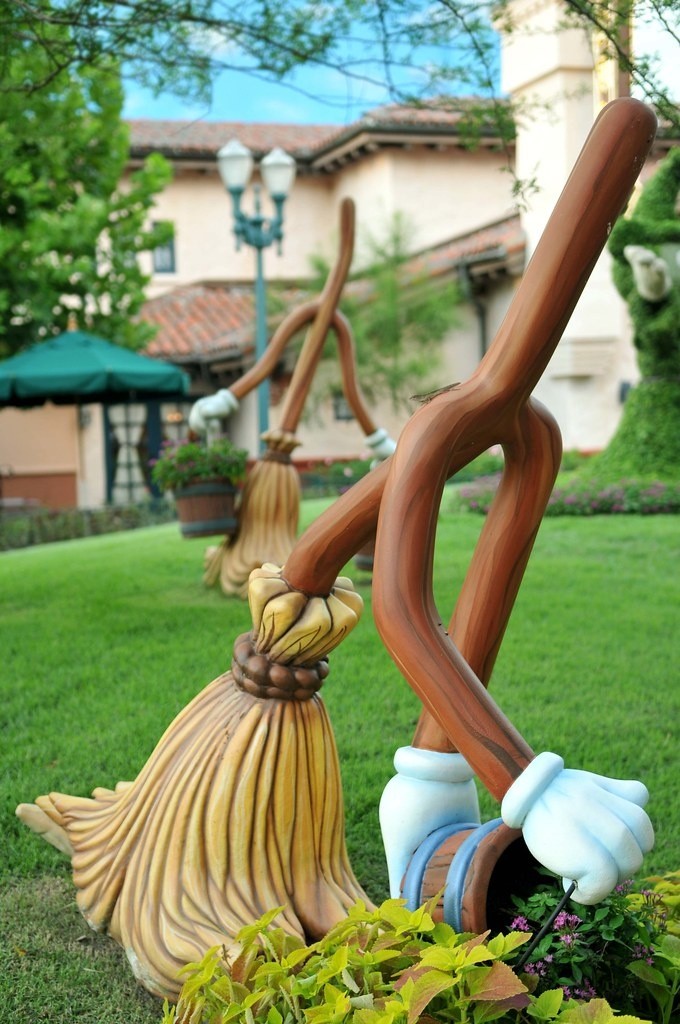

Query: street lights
216,139,295,458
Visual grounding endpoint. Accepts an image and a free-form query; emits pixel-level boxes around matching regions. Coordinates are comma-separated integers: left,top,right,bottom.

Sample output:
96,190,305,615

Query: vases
170,474,238,539
398,817,521,944
357,538,376,571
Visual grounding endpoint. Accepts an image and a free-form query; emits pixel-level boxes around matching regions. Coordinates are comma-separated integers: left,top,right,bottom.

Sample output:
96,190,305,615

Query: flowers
149,435,249,492
498,862,668,1005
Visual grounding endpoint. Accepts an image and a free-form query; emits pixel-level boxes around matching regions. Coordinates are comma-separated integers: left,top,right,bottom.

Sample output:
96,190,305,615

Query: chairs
0,465,40,513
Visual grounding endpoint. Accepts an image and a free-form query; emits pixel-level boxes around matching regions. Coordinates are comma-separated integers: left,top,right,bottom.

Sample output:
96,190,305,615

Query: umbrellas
0,329,191,479
